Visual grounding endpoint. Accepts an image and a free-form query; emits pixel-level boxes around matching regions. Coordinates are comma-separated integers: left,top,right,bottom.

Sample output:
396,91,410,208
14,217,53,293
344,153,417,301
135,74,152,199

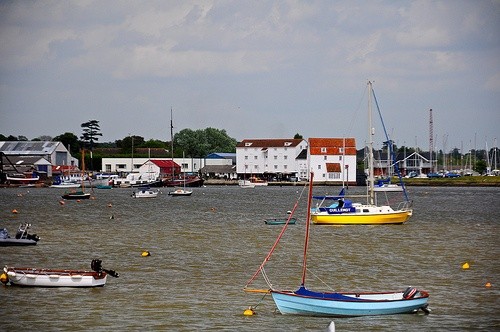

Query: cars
445,172,461,178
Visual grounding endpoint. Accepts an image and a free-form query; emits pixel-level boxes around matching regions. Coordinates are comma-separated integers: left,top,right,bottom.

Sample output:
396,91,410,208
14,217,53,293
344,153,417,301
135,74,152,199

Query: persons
337,198,345,208
286,175,288,181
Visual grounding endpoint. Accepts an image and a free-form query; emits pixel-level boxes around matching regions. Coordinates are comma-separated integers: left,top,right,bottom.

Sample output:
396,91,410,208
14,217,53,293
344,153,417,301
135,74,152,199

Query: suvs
427,172,443,178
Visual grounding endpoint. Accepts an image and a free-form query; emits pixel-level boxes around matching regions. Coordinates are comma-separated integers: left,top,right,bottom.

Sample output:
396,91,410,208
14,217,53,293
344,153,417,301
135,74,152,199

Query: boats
0,221,40,245
367,177,406,192
130,187,158,198
264,218,296,224
0,258,120,287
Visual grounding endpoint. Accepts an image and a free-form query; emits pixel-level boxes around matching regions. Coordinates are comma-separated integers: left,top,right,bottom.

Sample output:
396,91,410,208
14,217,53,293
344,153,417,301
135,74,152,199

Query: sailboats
242,170,431,318
168,150,193,195
61,149,91,199
309,78,414,225
